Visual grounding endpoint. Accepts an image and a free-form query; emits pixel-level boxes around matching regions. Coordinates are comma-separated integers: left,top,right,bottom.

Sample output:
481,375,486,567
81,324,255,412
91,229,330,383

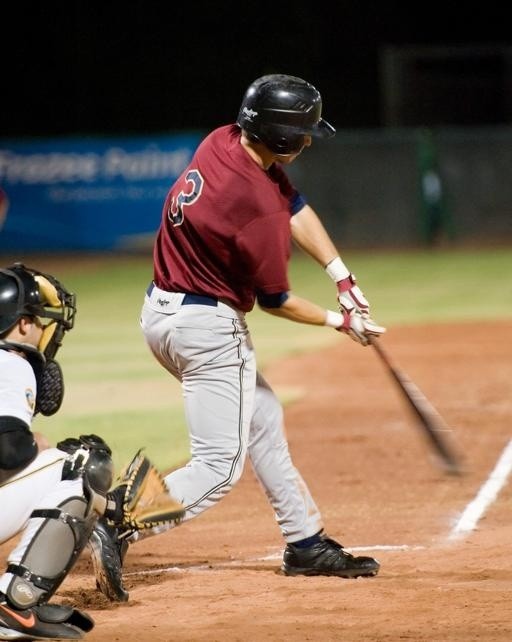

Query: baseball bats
368,333,468,470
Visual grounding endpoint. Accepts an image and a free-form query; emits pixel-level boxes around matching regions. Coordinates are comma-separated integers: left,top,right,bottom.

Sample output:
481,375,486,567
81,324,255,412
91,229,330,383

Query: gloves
324,256,385,347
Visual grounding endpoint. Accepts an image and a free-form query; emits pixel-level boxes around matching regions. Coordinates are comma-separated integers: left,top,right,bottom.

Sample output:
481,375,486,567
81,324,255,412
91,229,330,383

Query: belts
146,280,217,307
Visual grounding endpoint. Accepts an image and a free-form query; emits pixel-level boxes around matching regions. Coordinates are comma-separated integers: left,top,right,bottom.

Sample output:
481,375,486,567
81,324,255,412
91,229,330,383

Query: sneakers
84,518,129,601
0,592,82,641
282,532,379,579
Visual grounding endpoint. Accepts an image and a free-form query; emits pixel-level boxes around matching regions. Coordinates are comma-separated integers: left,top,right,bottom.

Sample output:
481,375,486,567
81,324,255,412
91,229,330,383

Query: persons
83,72,388,603
0,262,114,640
421,158,445,247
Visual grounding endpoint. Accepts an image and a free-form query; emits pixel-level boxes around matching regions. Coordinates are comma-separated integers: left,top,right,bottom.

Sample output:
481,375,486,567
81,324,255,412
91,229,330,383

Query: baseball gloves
108,454,186,529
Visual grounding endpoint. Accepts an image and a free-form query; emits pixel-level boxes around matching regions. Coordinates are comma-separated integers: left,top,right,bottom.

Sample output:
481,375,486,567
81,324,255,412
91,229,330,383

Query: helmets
0,262,75,361
237,74,336,155
